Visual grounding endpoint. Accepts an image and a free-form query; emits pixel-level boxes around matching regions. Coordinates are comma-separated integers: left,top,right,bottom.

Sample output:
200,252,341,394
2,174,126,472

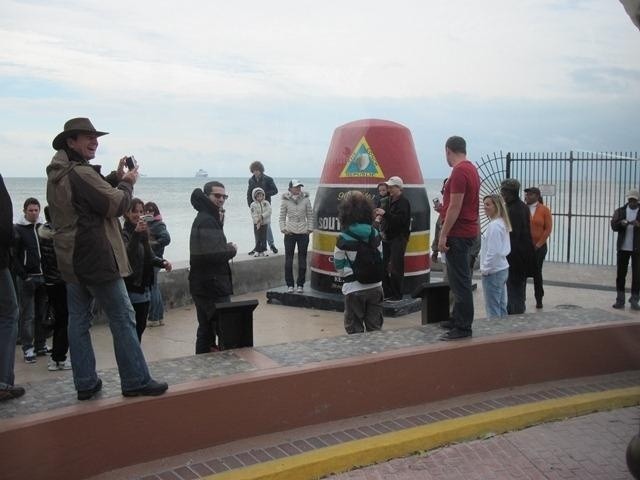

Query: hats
500,178,520,190
384,177,402,188
289,179,304,188
53,118,109,150
524,187,540,193
627,190,639,200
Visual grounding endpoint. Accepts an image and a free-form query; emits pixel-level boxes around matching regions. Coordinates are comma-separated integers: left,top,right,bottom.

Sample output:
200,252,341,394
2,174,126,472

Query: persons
45,117,169,401
481,191,511,317
250,188,271,259
333,193,385,337
0,174,28,403
499,178,534,316
277,179,316,292
610,190,640,311
428,178,454,309
246,160,280,256
187,180,238,355
522,186,553,311
10,195,171,372
374,182,388,224
434,135,482,341
380,174,412,301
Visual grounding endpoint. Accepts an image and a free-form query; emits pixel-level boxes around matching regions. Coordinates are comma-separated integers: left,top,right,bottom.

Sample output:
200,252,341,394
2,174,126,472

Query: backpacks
341,226,382,284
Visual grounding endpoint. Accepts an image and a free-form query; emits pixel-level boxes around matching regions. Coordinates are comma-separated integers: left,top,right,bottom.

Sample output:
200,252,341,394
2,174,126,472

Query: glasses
145,209,153,212
210,192,228,199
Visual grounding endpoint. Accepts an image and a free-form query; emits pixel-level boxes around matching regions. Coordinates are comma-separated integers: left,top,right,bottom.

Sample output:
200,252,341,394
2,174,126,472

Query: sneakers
248,245,278,256
386,295,404,302
296,286,303,293
536,303,543,309
440,327,472,340
631,302,640,310
613,301,624,307
441,318,456,329
288,286,294,293
1,320,168,398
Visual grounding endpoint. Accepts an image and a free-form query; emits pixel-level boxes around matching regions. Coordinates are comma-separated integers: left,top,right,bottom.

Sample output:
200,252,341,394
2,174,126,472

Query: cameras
139,214,153,224
126,156,138,172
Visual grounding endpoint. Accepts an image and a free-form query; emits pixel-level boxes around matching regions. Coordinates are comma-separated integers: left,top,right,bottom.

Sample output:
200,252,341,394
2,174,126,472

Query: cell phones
433,198,440,206
